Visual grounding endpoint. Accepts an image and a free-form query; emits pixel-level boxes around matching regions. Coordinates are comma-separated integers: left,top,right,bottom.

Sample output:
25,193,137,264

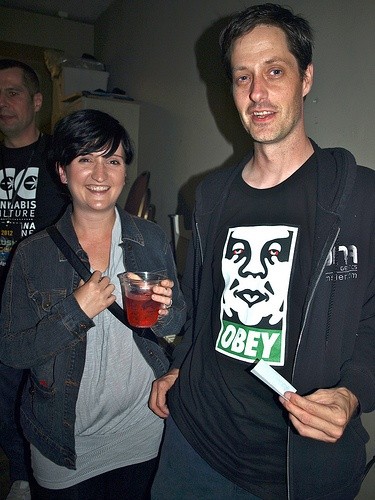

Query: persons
0,109,187,500
0,58,71,500
148,2,375,500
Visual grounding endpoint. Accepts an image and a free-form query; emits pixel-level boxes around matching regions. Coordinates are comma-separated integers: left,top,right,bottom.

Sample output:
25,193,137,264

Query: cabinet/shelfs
50,97,139,209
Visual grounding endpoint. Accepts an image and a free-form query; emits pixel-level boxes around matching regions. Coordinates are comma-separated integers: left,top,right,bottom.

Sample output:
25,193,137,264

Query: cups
120,272,168,328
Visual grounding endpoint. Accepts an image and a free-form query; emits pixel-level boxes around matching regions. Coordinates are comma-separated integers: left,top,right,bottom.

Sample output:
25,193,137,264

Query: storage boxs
54,67,108,100
